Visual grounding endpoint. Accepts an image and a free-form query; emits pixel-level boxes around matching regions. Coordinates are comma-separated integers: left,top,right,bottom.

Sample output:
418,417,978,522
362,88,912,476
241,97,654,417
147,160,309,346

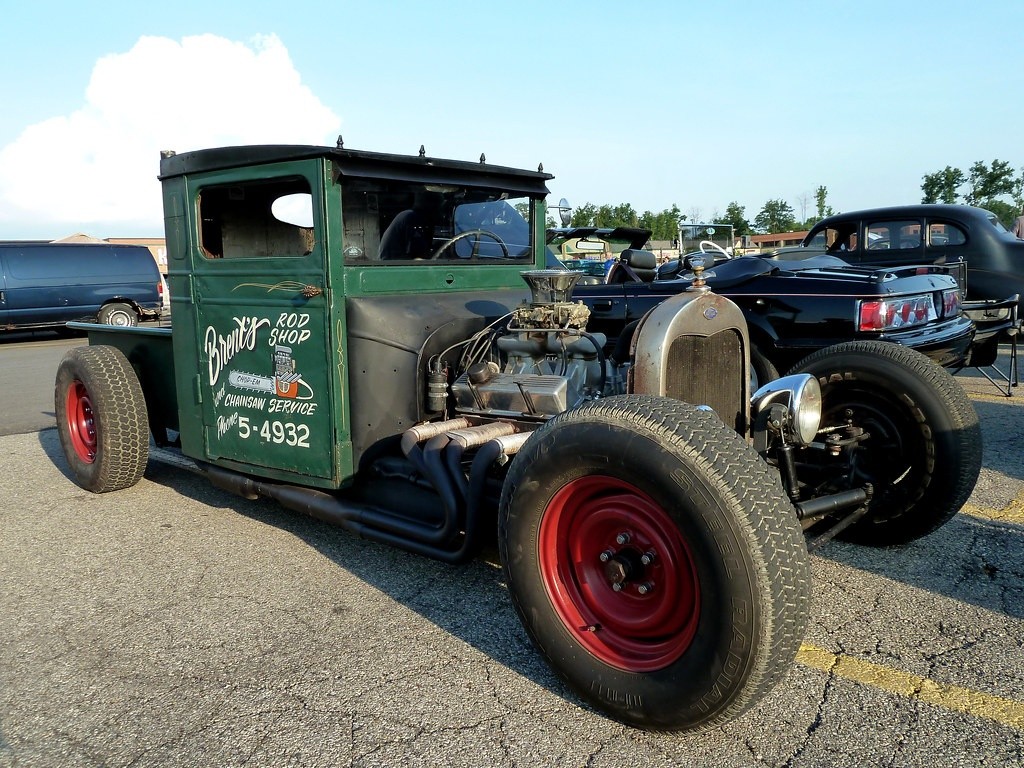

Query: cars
800,204,1024,346
869,233,948,250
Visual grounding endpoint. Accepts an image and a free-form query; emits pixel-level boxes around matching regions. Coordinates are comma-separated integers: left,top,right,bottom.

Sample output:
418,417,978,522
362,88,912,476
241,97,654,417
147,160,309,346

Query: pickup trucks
53,143,981,729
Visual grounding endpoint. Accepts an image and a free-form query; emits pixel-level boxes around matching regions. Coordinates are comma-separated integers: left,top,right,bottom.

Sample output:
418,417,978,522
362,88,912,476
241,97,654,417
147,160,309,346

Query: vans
0,242,164,337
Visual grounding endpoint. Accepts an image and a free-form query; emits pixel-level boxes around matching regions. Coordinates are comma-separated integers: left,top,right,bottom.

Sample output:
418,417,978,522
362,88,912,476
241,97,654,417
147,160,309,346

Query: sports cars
453,200,975,376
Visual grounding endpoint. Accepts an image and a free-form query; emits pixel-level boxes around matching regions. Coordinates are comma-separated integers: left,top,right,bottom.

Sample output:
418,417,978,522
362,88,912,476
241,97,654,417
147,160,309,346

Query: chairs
948,294,1024,398
604,249,657,284
